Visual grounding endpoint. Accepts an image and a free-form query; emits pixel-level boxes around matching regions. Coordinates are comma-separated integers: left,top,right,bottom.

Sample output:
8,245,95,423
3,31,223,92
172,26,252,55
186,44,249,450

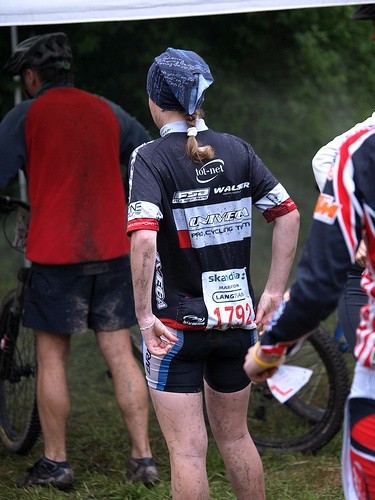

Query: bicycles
0,194,356,459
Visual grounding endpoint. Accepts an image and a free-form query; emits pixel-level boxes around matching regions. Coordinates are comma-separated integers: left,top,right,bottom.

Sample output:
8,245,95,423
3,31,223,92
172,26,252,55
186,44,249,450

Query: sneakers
126,458,158,484
15,458,75,490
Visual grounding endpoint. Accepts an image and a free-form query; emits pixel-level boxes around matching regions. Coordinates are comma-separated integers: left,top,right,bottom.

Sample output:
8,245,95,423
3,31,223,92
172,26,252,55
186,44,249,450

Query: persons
0,32,158,489
242,111,374,500
128,47,300,500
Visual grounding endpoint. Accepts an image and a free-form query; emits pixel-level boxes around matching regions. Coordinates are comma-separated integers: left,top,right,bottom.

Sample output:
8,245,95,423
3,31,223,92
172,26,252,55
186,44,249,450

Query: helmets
2,33,73,77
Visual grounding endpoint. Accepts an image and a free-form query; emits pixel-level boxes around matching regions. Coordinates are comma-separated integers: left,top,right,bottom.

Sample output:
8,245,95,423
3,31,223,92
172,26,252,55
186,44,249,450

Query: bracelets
253,339,286,366
140,314,157,331
